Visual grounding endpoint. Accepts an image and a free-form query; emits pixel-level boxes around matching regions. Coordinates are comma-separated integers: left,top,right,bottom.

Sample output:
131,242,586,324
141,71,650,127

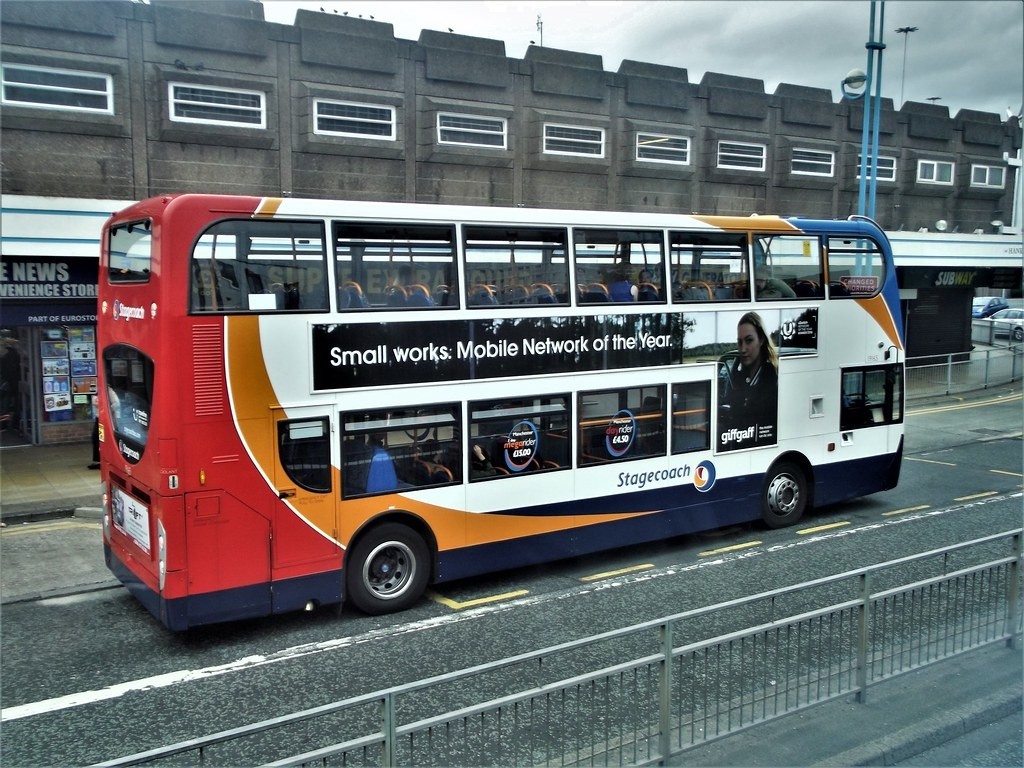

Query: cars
971,296,1009,318
981,307,1024,341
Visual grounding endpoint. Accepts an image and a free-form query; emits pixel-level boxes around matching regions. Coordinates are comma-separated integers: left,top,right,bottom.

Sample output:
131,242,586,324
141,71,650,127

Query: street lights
894,25,921,107
840,0,887,278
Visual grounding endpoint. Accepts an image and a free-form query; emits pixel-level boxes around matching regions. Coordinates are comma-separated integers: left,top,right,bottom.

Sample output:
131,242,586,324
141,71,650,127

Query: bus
95,194,906,633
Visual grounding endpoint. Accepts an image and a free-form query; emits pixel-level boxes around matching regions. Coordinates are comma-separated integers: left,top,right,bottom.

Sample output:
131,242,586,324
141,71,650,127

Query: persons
88,369,121,470
421,438,496,481
733,312,779,400
639,262,673,289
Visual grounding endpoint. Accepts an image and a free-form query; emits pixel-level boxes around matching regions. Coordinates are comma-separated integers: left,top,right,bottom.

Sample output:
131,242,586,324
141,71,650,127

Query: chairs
345,396,671,493
263,279,849,308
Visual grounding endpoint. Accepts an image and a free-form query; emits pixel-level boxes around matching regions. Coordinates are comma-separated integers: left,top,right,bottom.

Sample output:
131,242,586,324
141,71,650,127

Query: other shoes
88,463,100,469
